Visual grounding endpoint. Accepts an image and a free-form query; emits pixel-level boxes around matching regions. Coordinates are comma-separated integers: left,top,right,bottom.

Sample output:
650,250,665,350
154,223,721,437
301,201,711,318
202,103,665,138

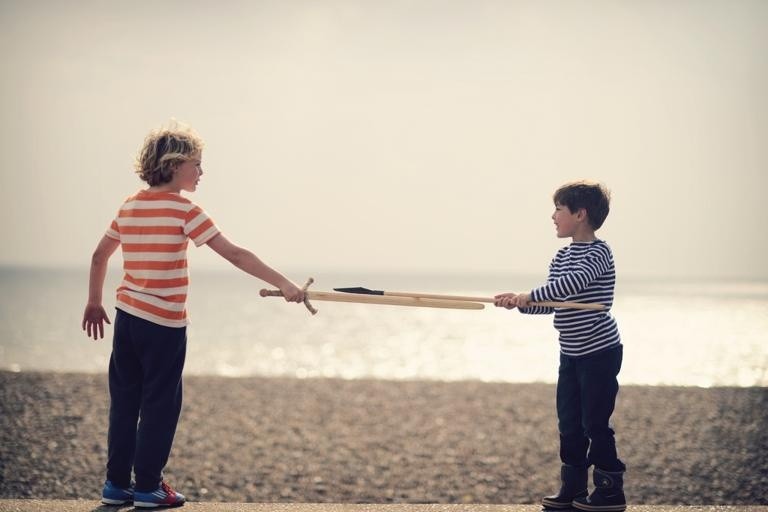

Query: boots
542,463,627,512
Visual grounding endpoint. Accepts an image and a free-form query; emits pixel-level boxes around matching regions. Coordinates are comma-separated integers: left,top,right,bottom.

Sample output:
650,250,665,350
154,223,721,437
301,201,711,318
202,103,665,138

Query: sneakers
102,474,186,508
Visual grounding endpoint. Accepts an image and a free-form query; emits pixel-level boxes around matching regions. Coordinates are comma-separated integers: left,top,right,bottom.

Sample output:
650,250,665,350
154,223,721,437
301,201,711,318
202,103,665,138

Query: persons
493,178,627,512
82,115,306,508
